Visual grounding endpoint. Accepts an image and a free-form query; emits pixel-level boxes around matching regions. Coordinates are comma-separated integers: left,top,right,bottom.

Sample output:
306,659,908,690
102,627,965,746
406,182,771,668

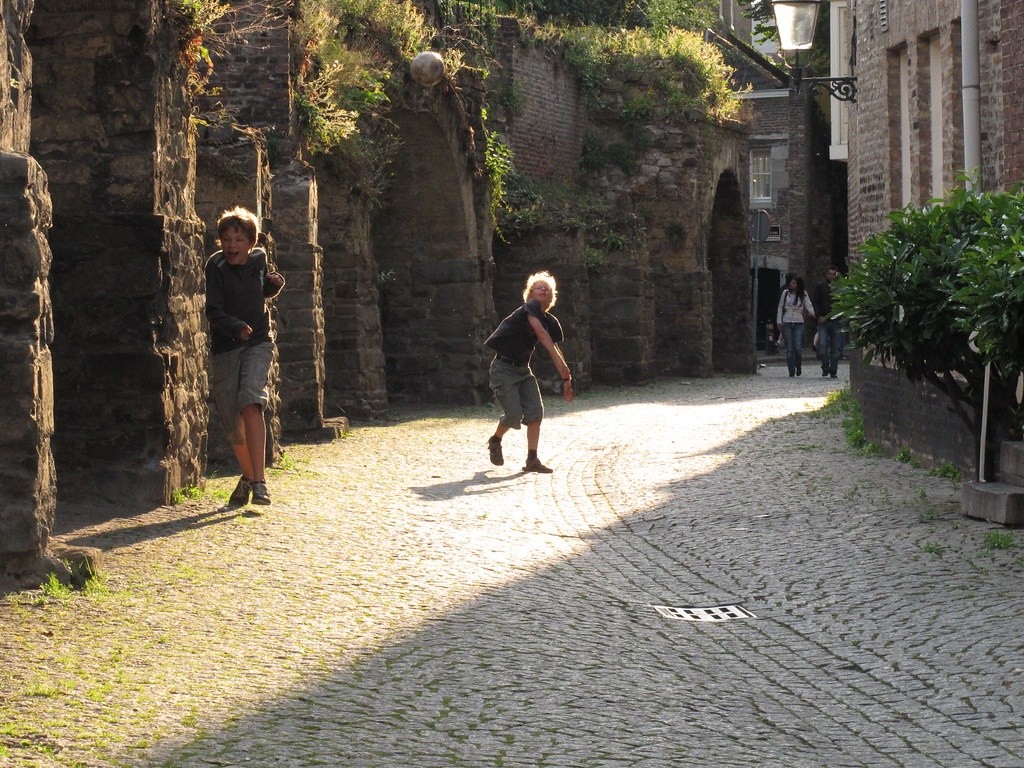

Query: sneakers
229,475,252,507
251,481,271,504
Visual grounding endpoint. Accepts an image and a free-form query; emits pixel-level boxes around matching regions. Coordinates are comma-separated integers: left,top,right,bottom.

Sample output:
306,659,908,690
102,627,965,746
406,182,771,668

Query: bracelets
564,378,569,381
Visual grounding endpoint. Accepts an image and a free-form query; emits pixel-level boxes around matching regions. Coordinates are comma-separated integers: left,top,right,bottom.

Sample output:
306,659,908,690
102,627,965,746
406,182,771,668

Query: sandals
522,458,553,473
488,437,504,466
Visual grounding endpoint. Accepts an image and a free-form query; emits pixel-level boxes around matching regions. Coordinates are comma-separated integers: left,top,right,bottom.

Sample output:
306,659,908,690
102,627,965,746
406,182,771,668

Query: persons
813,265,845,378
775,272,800,348
777,277,816,377
204,209,285,509
483,271,573,474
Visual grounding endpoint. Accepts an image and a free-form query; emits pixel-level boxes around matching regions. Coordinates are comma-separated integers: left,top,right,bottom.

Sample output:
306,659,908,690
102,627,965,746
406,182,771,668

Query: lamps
772,0,858,104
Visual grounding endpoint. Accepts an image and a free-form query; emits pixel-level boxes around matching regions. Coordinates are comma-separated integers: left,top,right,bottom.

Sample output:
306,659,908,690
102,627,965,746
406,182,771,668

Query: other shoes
797,368,801,376
789,372,794,377
822,370,828,376
832,373,837,378
